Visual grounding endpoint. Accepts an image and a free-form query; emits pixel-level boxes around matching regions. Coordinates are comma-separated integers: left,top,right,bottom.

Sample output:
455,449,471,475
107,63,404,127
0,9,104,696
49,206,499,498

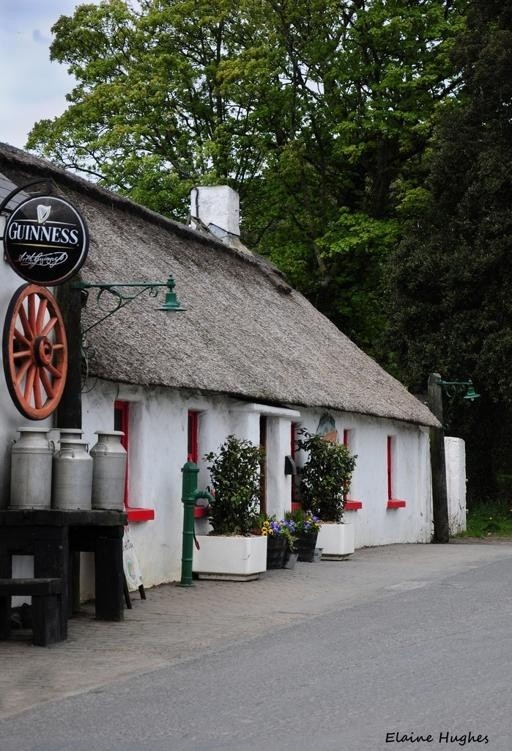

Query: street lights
54,272,187,612
426,373,479,542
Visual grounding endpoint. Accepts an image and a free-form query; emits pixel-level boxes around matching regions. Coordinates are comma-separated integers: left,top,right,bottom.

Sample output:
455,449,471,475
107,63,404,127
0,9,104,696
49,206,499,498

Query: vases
267,531,319,567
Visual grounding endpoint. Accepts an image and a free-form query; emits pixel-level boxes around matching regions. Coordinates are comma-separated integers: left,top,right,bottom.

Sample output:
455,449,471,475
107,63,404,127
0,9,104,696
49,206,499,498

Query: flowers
256,510,317,538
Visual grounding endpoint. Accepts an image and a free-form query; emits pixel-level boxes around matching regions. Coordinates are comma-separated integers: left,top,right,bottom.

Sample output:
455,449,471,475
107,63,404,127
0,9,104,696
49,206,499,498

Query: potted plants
293,425,355,562
190,437,267,581
